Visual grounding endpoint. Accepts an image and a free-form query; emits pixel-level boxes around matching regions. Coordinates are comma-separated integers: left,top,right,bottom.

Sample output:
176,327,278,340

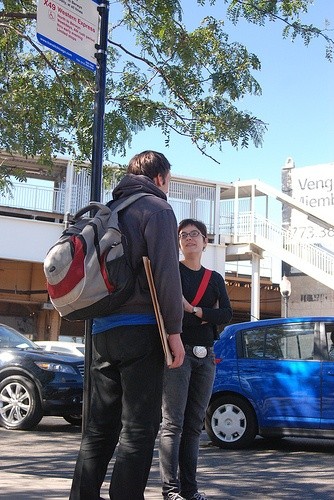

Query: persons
69,151,185,500
329,332,334,360
157,219,231,500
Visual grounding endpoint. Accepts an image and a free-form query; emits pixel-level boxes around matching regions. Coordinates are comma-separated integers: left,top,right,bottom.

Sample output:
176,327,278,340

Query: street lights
278,273,292,360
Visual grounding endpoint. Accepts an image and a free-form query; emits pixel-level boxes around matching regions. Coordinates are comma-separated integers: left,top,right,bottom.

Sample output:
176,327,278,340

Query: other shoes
186,492,208,500
164,492,186,500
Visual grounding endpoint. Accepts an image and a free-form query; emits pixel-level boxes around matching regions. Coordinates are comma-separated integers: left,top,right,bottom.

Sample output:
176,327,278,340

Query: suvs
0,323,85,430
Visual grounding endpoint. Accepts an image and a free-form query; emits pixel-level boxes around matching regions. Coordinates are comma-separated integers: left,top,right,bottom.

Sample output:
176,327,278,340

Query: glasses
178,230,205,238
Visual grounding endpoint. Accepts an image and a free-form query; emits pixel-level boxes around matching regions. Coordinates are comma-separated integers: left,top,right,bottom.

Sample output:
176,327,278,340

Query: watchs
192,307,198,314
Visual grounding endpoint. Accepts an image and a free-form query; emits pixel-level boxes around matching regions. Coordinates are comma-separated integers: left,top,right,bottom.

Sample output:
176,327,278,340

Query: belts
184,345,215,358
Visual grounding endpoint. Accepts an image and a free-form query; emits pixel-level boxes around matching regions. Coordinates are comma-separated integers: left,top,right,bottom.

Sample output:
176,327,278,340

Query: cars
204,316,334,449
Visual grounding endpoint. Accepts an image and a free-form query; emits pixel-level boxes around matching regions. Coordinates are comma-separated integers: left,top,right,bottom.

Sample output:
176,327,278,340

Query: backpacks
43,193,155,323
